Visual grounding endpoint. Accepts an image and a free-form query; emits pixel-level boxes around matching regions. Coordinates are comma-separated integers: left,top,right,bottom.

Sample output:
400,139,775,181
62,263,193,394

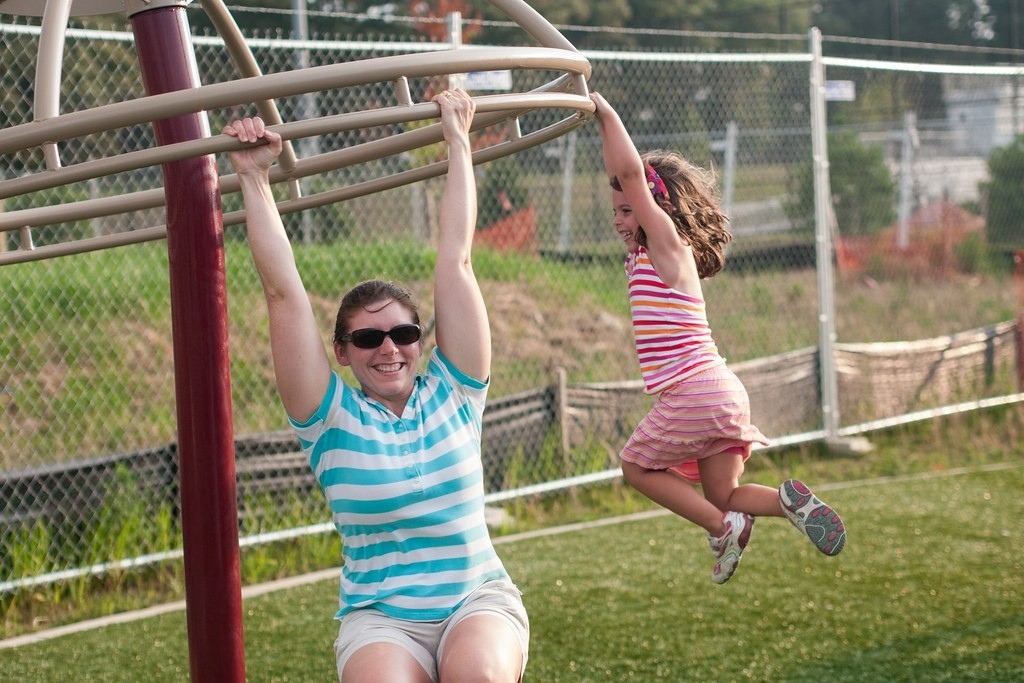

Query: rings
447,93,451,97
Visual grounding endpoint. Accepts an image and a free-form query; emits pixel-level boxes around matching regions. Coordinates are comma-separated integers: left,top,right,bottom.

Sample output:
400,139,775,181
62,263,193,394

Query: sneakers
708,511,757,585
777,478,846,558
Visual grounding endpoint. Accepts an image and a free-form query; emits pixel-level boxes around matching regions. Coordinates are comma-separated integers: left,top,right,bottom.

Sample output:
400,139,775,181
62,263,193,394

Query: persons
221,88,529,683
576,91,845,585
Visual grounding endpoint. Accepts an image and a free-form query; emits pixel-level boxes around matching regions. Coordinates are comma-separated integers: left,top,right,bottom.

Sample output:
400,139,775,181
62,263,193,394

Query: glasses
340,324,421,350
